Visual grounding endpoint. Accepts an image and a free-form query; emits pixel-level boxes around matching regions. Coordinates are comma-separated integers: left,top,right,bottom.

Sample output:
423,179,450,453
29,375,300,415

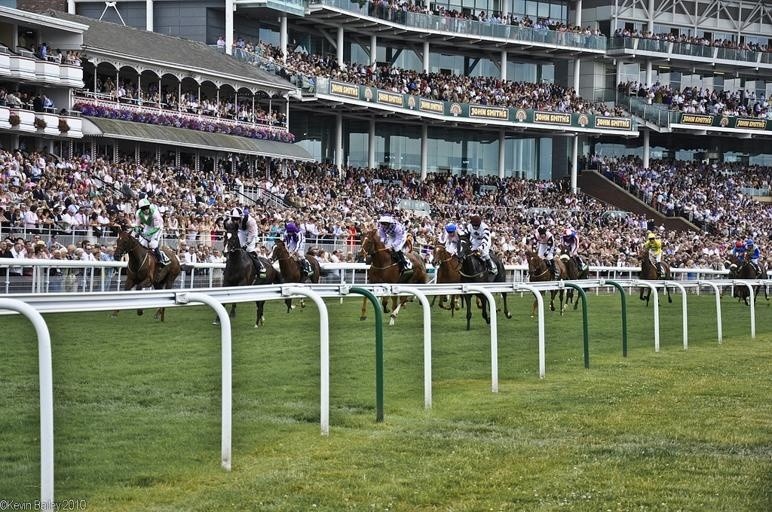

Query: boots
656,262,661,275
248,252,263,273
547,259,556,273
301,259,309,276
396,251,409,269
575,257,584,270
486,258,497,274
153,249,164,268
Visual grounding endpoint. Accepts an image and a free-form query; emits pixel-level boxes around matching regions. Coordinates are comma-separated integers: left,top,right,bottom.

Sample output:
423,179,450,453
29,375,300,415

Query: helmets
229,208,243,218
648,233,655,239
471,216,480,223
138,198,150,208
377,213,393,223
287,223,296,233
563,228,572,237
538,225,547,232
446,223,456,231
736,239,754,247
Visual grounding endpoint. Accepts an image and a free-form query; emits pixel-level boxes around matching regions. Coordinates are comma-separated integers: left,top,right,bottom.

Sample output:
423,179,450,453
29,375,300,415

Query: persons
0,0,772,295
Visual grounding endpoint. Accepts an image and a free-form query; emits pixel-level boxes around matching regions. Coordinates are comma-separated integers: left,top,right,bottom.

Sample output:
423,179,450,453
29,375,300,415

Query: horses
637,248,673,307
356,229,428,322
267,238,331,314
522,246,561,318
113,228,194,323
432,240,483,311
456,231,514,330
212,218,284,329
543,248,590,312
721,253,770,306
